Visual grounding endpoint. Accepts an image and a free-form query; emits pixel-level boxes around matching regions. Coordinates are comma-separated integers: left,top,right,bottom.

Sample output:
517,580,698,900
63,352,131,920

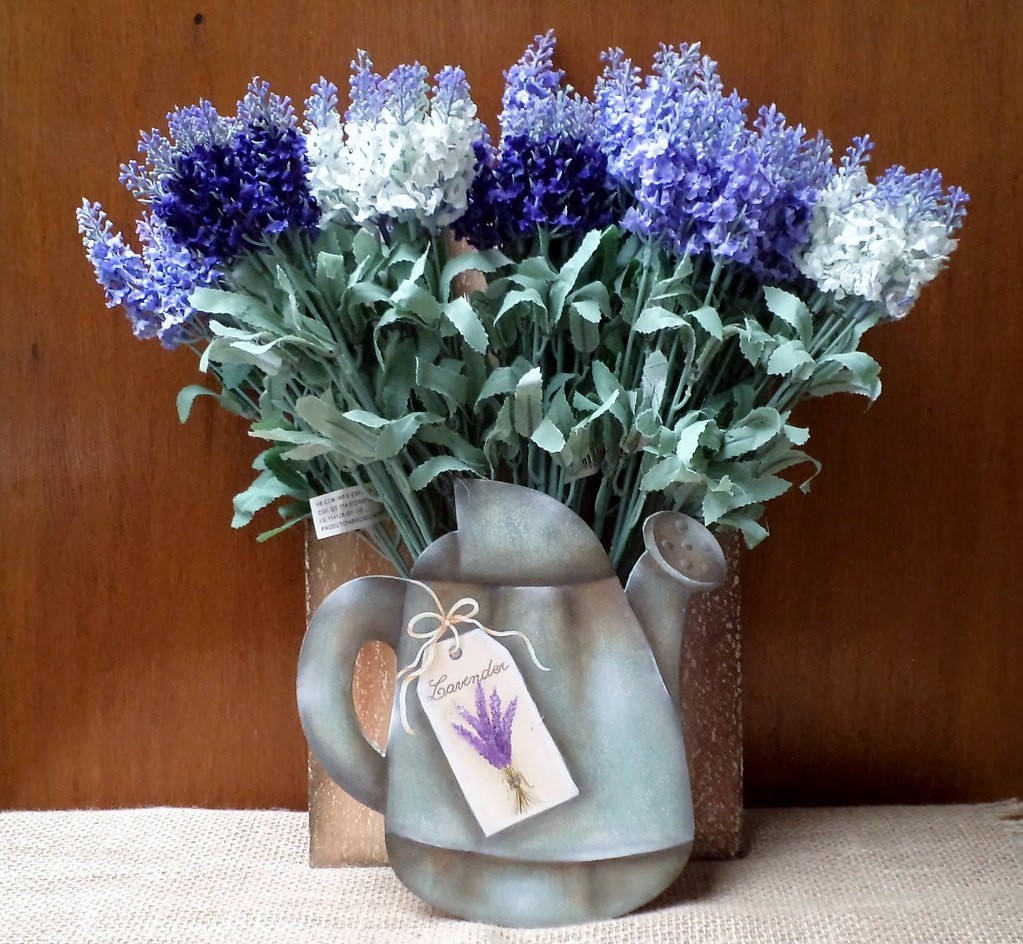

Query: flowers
66,28,972,592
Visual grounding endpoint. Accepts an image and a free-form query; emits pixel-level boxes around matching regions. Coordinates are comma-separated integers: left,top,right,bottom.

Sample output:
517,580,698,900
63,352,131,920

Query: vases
284,510,763,926
